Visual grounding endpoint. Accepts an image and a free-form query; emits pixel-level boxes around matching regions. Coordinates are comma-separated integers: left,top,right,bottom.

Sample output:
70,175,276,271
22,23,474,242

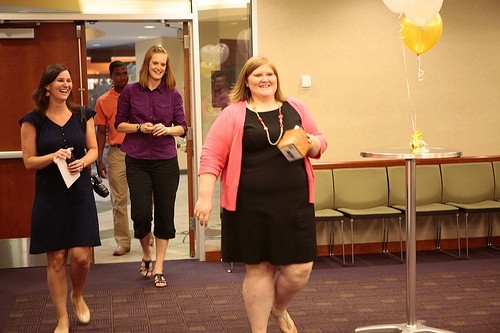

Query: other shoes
113,246,130,256
271,306,297,333
54,319,69,333
69,290,91,324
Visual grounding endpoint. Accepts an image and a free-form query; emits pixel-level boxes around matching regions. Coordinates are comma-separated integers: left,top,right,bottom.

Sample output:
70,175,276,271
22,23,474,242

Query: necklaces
251,100,284,147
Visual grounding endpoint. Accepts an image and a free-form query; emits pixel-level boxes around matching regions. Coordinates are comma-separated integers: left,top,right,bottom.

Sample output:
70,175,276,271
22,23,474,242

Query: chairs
221,161,500,273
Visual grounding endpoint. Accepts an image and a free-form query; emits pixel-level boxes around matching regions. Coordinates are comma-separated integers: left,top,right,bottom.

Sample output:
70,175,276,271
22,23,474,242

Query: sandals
139,258,154,278
154,273,167,288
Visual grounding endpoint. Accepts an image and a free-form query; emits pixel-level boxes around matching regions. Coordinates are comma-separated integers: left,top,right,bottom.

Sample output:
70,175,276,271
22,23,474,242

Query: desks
355,148,462,333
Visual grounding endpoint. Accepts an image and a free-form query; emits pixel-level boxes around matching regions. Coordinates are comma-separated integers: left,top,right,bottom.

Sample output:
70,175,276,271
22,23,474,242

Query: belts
112,144,121,148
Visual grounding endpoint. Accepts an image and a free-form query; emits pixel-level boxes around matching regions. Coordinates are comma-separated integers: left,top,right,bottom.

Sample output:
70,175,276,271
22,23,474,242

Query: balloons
382,0,444,28
399,12,444,57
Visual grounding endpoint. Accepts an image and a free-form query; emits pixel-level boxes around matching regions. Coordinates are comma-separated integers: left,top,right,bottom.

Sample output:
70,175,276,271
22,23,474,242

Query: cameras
89,176,109,198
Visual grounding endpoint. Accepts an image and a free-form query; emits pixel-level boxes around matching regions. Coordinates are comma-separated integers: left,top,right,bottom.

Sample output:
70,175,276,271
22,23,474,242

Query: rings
195,213,200,217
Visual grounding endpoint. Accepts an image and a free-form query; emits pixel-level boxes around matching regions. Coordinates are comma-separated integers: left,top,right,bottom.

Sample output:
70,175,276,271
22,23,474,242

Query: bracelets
137,124,142,132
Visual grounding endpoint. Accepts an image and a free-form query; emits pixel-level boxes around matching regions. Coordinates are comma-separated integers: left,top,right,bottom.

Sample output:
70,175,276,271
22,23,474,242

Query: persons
113,46,189,288
94,60,155,257
19,63,100,333
212,74,231,108
193,56,328,333
